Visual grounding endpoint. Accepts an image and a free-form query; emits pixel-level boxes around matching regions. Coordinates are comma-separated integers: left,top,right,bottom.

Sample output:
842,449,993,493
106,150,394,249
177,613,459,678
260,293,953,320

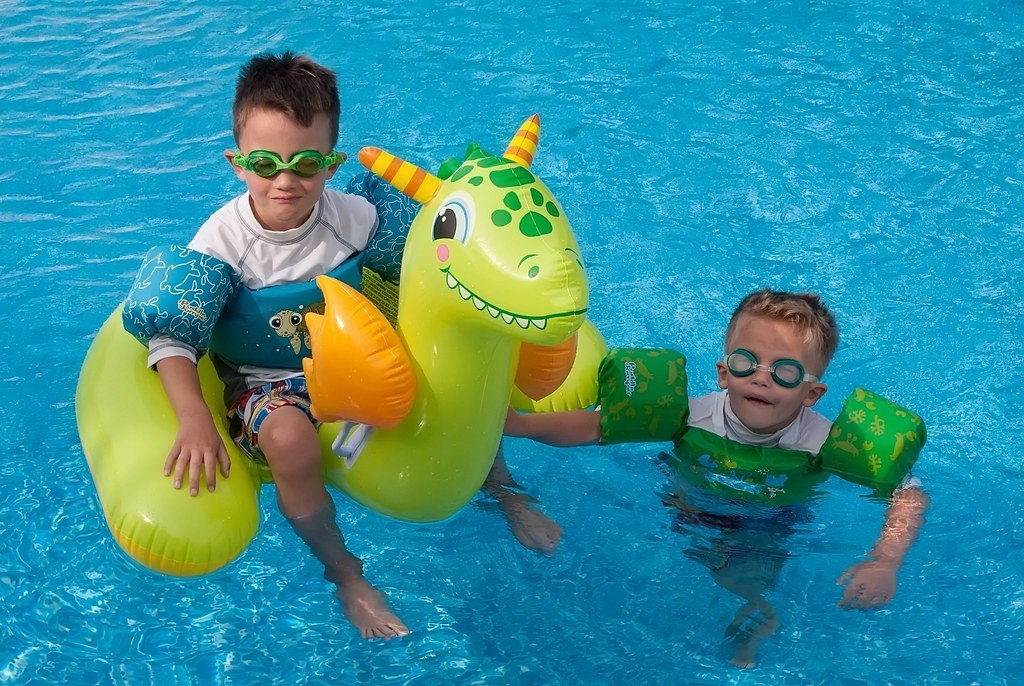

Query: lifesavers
593,346,930,522
69,112,615,580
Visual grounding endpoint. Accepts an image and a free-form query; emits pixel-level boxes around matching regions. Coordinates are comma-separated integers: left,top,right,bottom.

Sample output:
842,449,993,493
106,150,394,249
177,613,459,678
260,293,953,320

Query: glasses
233,148,345,177
723,348,818,387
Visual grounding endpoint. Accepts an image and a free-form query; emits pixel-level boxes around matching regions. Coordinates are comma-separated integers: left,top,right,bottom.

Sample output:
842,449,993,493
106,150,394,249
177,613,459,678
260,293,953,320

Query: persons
504,288,839,456
146,50,382,514
